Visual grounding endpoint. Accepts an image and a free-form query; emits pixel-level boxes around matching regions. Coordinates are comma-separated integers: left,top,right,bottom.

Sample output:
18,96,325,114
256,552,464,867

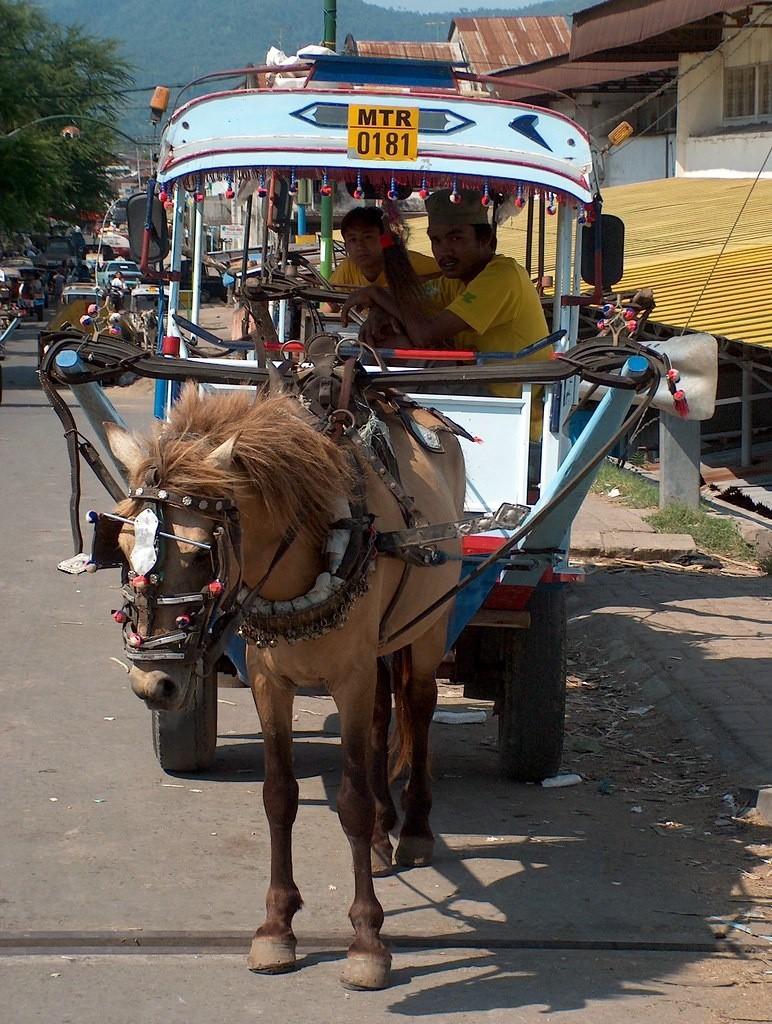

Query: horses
104,369,466,990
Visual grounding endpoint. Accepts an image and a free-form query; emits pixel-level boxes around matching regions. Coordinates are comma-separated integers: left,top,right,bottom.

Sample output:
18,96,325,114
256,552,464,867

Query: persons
17,222,92,312
184,227,189,246
340,188,557,441
314,207,444,312
109,272,127,295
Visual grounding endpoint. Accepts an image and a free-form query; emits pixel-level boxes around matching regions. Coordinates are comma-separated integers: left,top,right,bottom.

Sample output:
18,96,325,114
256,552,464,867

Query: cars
63,282,105,303
95,260,145,291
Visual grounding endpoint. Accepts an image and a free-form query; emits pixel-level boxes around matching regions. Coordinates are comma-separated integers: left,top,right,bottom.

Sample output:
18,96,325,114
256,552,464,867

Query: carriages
36,48,696,991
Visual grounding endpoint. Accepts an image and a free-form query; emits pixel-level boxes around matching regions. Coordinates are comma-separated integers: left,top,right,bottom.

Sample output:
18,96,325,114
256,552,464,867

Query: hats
425,188,490,227
